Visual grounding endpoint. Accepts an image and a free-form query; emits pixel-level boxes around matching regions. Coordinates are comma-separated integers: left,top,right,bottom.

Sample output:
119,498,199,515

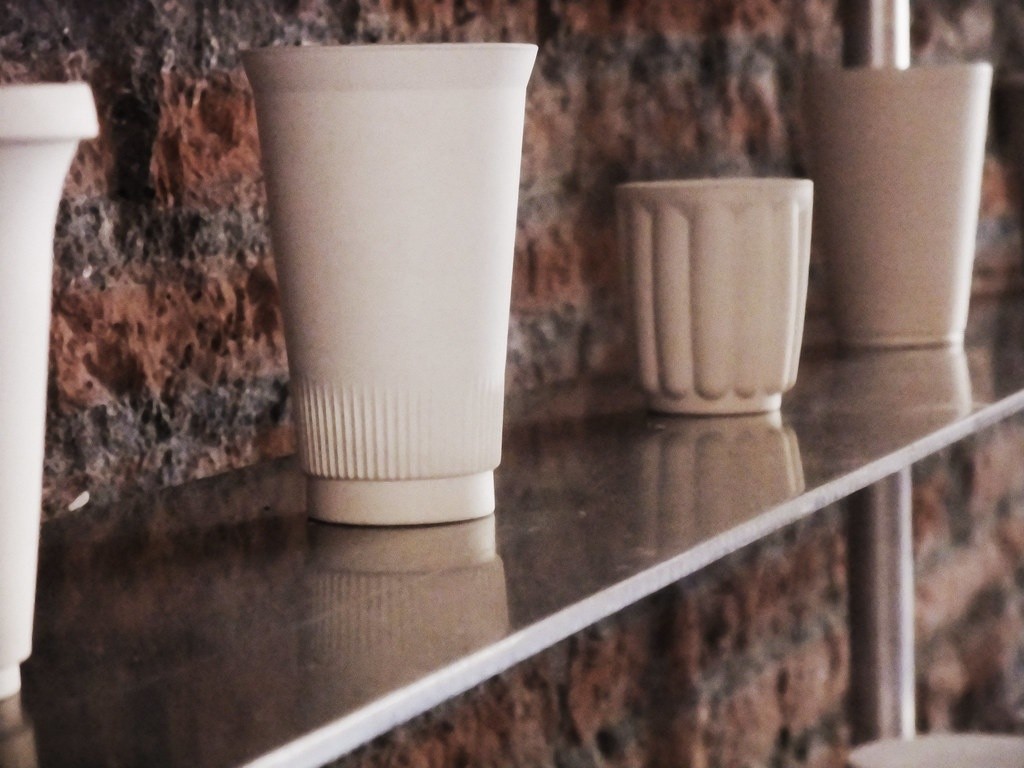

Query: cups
615,171,821,417
842,735,1020,766
0,73,104,700
800,52,994,353
234,41,544,532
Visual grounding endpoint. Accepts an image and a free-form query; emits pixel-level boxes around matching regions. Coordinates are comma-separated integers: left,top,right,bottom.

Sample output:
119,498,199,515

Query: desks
3,295,1024,766
